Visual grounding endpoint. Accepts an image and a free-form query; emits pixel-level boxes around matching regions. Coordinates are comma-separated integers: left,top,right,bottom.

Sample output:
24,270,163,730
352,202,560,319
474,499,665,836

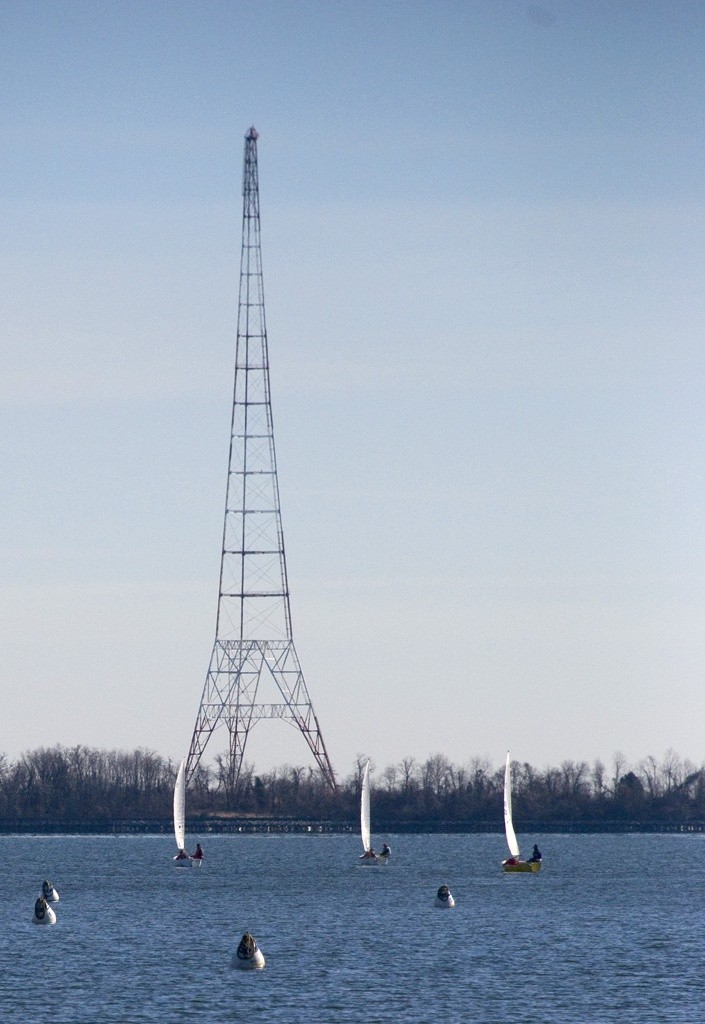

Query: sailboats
358,761,389,866
500,750,542,873
173,755,203,868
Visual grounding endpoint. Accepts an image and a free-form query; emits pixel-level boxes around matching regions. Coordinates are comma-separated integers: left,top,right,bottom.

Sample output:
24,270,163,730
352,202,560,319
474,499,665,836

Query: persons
526,844,542,863
379,843,392,858
191,843,204,859
